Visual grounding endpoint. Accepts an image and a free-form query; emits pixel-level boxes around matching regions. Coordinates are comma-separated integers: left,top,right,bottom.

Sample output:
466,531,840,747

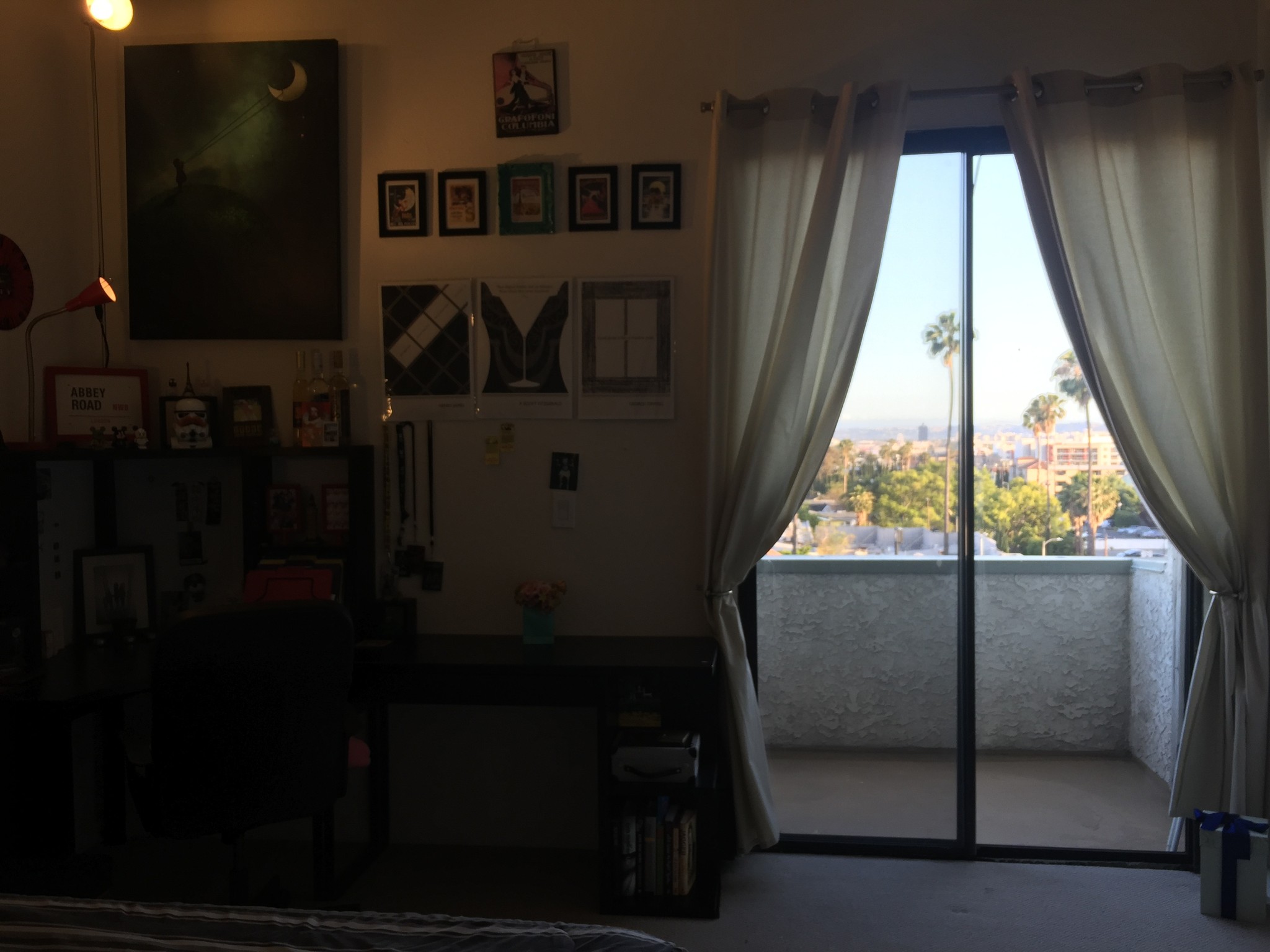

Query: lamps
22,276,118,441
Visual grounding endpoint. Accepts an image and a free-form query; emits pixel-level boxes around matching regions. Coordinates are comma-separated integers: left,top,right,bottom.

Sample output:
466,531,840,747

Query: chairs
129,599,356,904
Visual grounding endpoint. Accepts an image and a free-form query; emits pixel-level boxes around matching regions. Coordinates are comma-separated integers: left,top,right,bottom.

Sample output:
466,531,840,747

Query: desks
1,624,728,918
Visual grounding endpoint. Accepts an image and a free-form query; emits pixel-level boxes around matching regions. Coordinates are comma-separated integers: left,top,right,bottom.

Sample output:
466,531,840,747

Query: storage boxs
1192,807,1270,924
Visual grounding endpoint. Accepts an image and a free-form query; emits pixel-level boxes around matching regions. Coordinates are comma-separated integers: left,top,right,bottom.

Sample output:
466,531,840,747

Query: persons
272,492,297,527
105,583,126,606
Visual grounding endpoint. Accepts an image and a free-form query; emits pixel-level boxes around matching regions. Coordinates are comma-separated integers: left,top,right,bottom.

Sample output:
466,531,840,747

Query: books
616,805,701,898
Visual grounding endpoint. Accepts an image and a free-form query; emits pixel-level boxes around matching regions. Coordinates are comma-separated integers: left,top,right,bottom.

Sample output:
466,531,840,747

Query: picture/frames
321,482,349,533
497,161,557,234
492,50,561,138
566,165,618,232
43,364,151,451
437,171,487,237
377,171,428,238
224,383,277,450
159,395,220,451
264,483,303,543
629,163,681,229
72,543,157,640
